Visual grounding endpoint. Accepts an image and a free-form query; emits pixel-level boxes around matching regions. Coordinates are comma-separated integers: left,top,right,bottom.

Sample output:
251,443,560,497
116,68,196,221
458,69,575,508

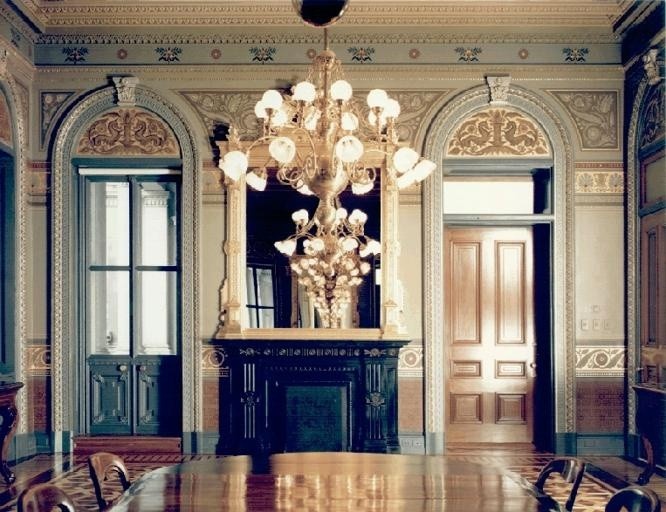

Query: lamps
218,28,441,224
273,170,383,329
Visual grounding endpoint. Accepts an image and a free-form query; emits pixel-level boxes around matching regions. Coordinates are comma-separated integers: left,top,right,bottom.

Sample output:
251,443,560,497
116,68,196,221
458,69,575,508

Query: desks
95,451,564,512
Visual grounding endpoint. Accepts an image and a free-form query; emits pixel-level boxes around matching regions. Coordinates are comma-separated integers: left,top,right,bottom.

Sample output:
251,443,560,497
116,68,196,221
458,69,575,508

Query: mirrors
212,140,404,340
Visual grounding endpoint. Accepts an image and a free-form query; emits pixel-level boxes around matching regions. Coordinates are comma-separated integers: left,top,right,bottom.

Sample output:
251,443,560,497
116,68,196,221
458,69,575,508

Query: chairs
536,458,661,511
17,452,130,512
